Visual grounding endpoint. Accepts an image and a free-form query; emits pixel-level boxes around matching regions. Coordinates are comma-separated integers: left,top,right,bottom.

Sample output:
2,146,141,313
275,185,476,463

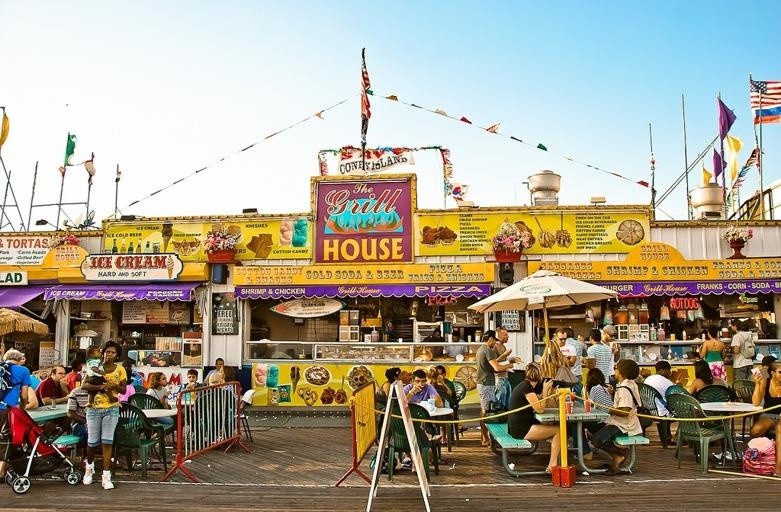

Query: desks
530,397,612,477
9,400,75,423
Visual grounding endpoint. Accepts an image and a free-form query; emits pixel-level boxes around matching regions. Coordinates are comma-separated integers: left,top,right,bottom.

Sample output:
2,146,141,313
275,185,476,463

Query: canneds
152,241,161,254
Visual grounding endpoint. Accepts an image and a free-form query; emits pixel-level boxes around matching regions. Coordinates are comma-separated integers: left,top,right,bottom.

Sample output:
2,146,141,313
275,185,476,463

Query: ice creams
166,254,174,279
290,366,301,394
161,219,173,253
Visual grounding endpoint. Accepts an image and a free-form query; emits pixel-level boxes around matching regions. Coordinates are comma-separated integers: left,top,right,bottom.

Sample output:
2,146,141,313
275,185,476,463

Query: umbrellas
465,268,621,381
1,309,49,352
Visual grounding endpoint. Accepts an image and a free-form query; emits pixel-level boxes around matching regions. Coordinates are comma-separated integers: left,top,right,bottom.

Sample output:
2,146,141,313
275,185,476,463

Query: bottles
468,335,472,341
168,384,191,403
219,366,225,378
448,335,453,341
674,352,680,360
584,400,592,411
683,331,687,339
52,396,57,408
399,338,403,342
475,331,483,342
718,321,723,337
103,237,161,254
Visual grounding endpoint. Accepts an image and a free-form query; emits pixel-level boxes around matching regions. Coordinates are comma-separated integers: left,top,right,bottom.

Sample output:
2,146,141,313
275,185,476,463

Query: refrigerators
120,327,167,350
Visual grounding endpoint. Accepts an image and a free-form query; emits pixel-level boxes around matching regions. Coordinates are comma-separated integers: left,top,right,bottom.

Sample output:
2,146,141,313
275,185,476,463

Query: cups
428,399,436,411
703,334,706,340
672,334,676,340
299,354,306,358
566,402,574,414
752,367,763,380
570,392,577,404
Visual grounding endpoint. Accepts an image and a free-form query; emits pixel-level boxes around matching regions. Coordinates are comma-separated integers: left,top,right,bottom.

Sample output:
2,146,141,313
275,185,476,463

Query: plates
346,366,374,391
304,365,332,387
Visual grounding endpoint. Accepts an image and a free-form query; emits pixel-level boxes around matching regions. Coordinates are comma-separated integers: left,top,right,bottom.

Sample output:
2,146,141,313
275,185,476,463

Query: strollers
0,399,82,495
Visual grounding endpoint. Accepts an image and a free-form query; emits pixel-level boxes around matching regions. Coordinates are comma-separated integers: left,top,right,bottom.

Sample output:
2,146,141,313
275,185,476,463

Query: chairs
638,380,764,476
111,393,222,481
385,380,466,481
228,388,255,445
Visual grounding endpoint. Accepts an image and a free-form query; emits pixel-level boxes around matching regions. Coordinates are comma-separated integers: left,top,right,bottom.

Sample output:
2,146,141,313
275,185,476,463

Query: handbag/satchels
484,401,508,424
370,454,397,474
638,406,653,427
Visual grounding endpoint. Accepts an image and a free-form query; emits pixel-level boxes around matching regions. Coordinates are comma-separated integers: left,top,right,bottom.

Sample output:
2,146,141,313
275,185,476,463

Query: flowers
722,225,754,245
50,230,83,249
490,222,532,253
202,228,243,253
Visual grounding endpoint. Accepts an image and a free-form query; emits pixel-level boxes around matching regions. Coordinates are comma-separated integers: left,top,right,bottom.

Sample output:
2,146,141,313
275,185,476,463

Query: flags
360,46,373,136
82,151,98,187
750,75,781,126
62,134,84,170
1,110,10,153
444,180,469,199
115,165,122,183
702,97,739,185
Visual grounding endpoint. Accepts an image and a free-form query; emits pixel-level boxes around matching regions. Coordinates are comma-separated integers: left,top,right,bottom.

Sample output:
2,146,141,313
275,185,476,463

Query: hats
484,330,500,341
604,325,617,335
762,356,778,366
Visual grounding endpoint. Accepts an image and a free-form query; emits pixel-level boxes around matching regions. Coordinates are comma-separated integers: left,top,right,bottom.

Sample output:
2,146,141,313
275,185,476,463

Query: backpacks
0,362,20,401
737,332,755,359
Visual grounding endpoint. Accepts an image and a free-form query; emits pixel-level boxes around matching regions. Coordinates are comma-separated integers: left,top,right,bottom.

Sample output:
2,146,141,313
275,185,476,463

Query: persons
146,372,174,439
645,360,679,444
554,324,641,476
1,341,142,489
474,330,515,446
376,328,466,473
686,321,781,477
178,358,235,442
509,362,561,474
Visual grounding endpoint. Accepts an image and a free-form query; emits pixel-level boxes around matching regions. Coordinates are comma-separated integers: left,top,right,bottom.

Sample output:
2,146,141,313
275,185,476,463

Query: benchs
611,431,652,476
37,433,87,465
483,421,535,479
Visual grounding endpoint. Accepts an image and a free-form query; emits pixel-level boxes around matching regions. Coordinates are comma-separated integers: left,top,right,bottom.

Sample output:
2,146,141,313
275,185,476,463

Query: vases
726,239,747,261
205,248,240,266
494,245,525,265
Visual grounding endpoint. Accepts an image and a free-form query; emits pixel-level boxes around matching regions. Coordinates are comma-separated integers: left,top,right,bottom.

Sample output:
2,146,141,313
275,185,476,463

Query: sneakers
102,475,114,489
401,458,411,467
83,468,94,485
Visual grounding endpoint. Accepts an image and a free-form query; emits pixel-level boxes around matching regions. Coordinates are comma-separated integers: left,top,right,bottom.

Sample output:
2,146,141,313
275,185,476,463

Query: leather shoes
545,448,628,473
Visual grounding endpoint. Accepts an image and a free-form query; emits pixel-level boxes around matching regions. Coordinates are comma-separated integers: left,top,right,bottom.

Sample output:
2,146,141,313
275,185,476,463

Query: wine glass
507,356,517,373
